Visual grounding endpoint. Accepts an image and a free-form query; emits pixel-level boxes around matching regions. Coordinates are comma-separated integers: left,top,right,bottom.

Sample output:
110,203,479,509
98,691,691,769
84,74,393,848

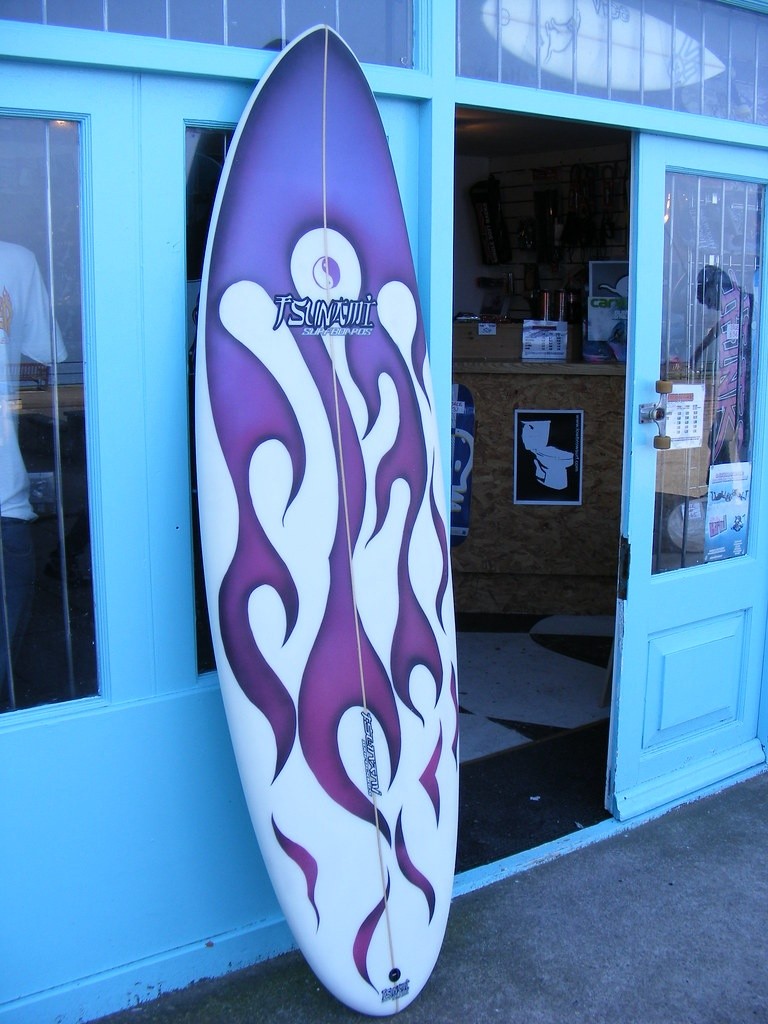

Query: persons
690,265,751,463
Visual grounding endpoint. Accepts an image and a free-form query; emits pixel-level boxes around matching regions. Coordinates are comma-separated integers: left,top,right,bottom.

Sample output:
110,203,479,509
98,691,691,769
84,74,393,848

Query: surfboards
195,19,467,1020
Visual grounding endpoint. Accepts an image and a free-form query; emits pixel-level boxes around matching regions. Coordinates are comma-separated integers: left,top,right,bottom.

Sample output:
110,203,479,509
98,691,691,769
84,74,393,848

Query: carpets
455,716,614,873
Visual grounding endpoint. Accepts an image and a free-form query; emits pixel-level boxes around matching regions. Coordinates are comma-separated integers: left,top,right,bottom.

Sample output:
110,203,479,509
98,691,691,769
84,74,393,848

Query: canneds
537,288,576,323
